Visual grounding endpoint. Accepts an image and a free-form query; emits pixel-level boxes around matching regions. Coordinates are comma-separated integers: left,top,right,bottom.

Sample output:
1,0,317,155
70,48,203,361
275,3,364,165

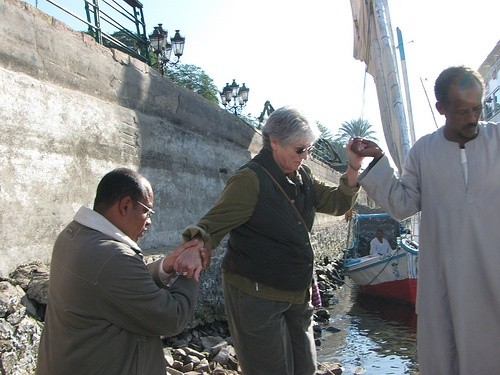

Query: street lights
149,24,186,75
219,79,249,116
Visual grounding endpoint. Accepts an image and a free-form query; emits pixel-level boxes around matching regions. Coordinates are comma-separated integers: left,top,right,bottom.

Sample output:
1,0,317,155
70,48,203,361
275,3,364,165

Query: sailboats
343,1,418,306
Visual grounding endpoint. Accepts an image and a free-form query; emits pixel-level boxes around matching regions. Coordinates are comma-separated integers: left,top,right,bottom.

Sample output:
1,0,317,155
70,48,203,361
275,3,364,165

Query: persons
36,168,212,375
173,106,367,375
350,65,500,375
369,228,393,257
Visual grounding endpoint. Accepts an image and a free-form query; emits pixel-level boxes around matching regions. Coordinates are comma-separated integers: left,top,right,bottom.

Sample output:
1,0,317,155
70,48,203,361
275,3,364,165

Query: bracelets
347,159,361,171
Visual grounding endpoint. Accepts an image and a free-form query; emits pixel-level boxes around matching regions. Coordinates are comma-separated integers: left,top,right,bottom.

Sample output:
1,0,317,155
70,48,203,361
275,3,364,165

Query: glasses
283,140,313,154
135,200,156,218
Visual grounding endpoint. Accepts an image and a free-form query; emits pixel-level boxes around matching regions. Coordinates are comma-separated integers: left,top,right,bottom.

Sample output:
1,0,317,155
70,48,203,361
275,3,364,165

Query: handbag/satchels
311,272,322,307
